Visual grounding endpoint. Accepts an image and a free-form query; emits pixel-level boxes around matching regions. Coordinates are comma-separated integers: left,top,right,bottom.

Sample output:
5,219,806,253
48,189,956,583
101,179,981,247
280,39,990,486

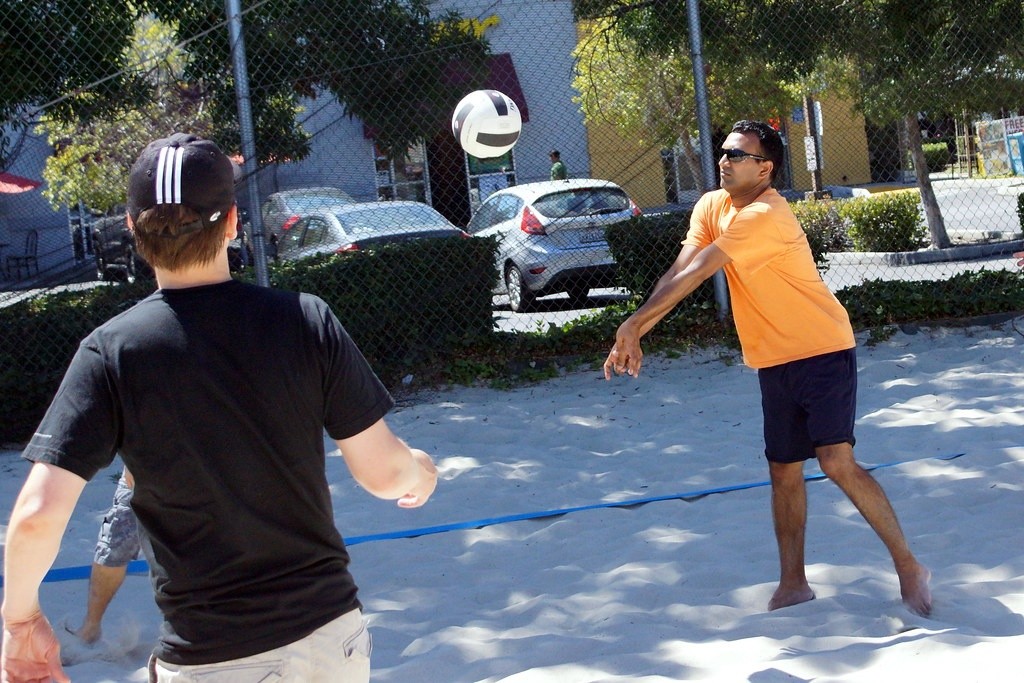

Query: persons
547,149,566,180
75,466,141,643
604,119,932,617
0,134,438,683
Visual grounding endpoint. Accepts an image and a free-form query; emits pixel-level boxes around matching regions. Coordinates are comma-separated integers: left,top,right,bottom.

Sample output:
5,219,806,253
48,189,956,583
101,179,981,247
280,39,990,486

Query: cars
463,178,642,313
271,201,475,266
90,198,243,285
261,186,354,247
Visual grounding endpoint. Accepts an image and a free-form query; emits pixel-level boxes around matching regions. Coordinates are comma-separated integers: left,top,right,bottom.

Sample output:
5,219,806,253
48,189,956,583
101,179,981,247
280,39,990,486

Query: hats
127,133,244,238
547,150,560,157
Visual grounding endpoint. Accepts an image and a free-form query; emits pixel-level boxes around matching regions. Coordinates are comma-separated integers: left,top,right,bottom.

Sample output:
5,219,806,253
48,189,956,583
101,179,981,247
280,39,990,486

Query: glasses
718,147,769,162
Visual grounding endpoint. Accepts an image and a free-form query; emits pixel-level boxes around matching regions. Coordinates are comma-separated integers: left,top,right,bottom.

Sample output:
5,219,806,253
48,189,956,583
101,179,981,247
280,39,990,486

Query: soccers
451,89,523,160
